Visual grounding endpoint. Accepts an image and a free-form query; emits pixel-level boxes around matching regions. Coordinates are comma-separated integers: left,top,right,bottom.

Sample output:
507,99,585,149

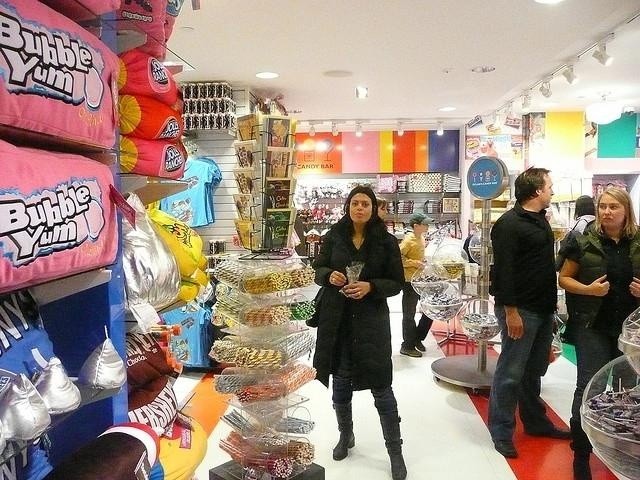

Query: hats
409,212,435,226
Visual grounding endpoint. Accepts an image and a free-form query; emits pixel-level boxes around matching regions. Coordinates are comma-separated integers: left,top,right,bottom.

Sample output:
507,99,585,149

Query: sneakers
400,340,427,357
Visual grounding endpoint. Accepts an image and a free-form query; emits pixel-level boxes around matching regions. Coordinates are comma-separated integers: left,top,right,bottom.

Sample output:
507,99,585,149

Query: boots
570,417,593,480
380,412,407,480
333,400,355,461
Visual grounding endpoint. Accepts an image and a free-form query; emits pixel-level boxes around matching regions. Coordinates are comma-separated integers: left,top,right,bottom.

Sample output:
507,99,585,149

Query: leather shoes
526,428,571,440
492,439,518,457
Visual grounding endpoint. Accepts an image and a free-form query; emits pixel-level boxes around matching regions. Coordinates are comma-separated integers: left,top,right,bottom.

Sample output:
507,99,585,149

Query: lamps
308,120,444,137
496,31,615,115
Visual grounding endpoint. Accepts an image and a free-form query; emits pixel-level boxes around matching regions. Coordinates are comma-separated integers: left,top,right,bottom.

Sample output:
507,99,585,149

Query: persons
489,165,571,459
560,194,596,345
399,212,434,359
313,186,407,480
463,222,482,267
373,194,389,230
557,187,640,480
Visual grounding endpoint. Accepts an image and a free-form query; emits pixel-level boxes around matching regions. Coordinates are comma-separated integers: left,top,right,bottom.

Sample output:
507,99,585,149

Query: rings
331,278,335,282
357,292,360,296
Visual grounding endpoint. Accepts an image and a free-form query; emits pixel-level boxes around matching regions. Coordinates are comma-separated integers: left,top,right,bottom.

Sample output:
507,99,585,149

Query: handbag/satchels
306,286,326,327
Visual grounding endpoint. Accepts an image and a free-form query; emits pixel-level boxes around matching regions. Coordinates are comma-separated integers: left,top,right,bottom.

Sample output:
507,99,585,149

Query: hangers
183,301,204,312
181,140,210,160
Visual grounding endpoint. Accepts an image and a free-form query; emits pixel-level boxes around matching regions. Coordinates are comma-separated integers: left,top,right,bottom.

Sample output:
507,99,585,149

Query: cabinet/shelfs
296,191,463,261
1,10,206,480
